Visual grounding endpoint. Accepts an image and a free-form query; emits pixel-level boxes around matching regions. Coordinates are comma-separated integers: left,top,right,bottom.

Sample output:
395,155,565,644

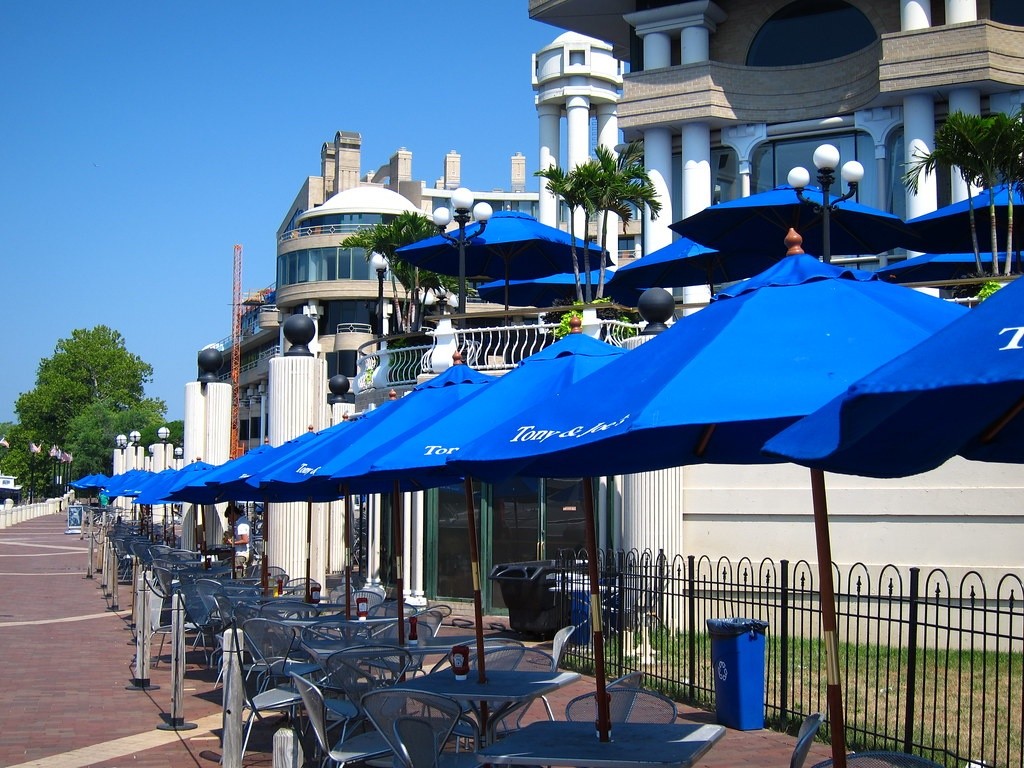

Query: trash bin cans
488,560,568,635
546,571,620,645
707,617,768,731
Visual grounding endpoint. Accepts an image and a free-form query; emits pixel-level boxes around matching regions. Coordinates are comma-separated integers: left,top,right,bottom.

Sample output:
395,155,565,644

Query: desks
477,720,726,768
279,614,411,653
301,635,477,716
393,668,582,746
251,602,360,649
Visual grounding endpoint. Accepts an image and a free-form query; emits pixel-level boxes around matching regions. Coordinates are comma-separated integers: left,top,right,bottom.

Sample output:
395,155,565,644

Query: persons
99,488,109,508
224,506,252,579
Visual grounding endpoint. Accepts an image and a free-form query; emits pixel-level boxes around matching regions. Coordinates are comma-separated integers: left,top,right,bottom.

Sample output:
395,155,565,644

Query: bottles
311,587,320,607
356,598,368,621
207,558,213,571
593,693,612,739
408,617,418,644
237,566,244,579
268,573,283,598
452,645,469,681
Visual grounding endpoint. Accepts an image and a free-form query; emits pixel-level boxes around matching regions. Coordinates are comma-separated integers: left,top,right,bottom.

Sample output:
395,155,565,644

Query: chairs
90,515,945,768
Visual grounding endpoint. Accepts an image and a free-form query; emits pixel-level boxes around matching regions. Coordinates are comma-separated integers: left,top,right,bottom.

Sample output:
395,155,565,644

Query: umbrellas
396,205,615,348
616,236,745,297
329,313,631,742
900,182,1024,254
877,251,1024,291
762,271,1024,478
448,228,972,768
70,351,500,749
478,268,649,308
668,183,909,257
